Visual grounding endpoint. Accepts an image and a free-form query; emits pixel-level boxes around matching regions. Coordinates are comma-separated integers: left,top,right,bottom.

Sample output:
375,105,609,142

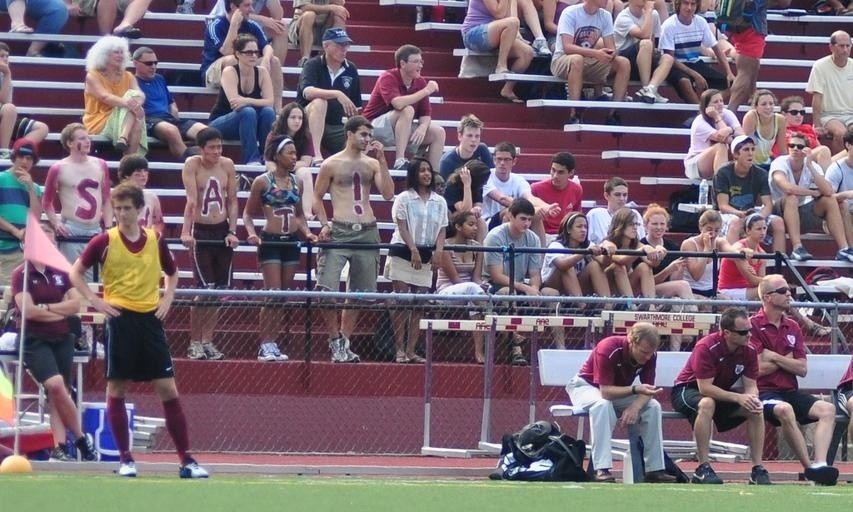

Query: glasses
333,42,351,48
785,109,806,116
766,287,792,294
237,48,261,56
724,328,753,336
787,143,805,149
832,43,851,48
137,60,159,66
624,221,641,227
405,59,425,64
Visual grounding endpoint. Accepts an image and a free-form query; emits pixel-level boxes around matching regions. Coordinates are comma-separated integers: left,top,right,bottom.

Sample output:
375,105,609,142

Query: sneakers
48,446,79,464
186,341,208,360
655,93,670,103
119,462,137,476
531,38,553,58
175,4,194,15
802,463,840,485
273,343,289,361
691,461,723,484
344,337,361,362
179,458,210,478
634,85,656,104
606,118,622,125
328,336,354,363
71,431,102,462
201,342,225,360
257,343,276,362
392,157,411,182
245,161,263,166
560,114,581,126
834,245,853,264
748,464,772,485
790,243,814,261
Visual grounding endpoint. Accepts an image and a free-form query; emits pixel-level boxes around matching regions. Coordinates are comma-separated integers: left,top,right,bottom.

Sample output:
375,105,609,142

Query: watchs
227,229,236,236
632,385,637,395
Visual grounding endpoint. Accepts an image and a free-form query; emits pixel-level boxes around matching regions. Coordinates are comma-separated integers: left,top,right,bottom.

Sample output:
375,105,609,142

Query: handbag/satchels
487,419,588,484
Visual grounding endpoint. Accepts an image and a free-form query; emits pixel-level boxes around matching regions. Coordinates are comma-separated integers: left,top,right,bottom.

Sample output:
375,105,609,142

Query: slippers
495,70,515,74
498,94,525,104
310,158,324,168
804,323,833,338
408,356,427,364
8,23,34,33
25,52,44,57
395,352,411,363
113,25,143,39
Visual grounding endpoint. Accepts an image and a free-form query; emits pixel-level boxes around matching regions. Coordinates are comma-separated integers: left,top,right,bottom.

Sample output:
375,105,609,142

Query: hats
730,135,754,153
321,27,353,43
9,137,40,164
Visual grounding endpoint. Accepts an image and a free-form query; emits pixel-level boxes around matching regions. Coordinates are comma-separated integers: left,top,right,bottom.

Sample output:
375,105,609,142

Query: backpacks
496,422,585,480
667,182,704,231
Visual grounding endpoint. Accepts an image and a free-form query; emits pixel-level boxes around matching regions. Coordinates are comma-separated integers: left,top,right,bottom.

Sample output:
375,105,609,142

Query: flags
22,212,72,272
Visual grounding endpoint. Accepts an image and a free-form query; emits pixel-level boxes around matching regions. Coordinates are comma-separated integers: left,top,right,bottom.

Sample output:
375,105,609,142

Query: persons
682,206,754,308
532,151,583,245
773,97,819,158
243,134,319,361
461,0,535,104
614,0,675,104
444,159,487,277
834,338,853,446
43,122,113,282
0,40,47,148
534,0,585,55
716,0,767,112
768,132,853,262
11,224,101,463
440,115,496,179
684,89,742,181
110,154,164,234
70,1,152,39
718,207,833,336
211,33,277,166
181,128,239,358
716,135,788,259
585,176,647,246
265,103,315,223
744,89,786,170
633,204,697,350
484,197,566,365
747,275,836,484
660,0,737,104
541,211,615,312
669,305,771,484
697,22,741,62
201,0,284,116
70,182,208,479
481,141,561,222
289,0,351,66
131,46,210,159
436,210,527,364
566,322,679,483
806,30,853,143
298,27,363,166
551,0,631,127
767,0,853,17
822,126,853,254
507,0,549,54
1,138,43,288
314,116,394,363
364,46,446,183
430,172,448,196
383,156,449,363
601,208,667,311
4,1,68,58
84,35,146,157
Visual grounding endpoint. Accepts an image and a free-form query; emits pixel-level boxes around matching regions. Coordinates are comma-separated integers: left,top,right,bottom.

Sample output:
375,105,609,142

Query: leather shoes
591,469,616,483
509,349,528,366
643,470,678,483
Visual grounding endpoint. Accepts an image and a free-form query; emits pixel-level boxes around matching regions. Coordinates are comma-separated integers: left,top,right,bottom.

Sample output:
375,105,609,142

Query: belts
260,233,295,242
331,219,377,232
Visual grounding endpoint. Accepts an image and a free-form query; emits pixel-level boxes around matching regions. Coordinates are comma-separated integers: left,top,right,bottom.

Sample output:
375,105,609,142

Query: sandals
511,332,531,346
116,136,129,152
475,353,486,365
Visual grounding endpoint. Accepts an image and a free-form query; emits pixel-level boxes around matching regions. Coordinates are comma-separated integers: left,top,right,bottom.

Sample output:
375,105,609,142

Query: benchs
539,349,852,484
